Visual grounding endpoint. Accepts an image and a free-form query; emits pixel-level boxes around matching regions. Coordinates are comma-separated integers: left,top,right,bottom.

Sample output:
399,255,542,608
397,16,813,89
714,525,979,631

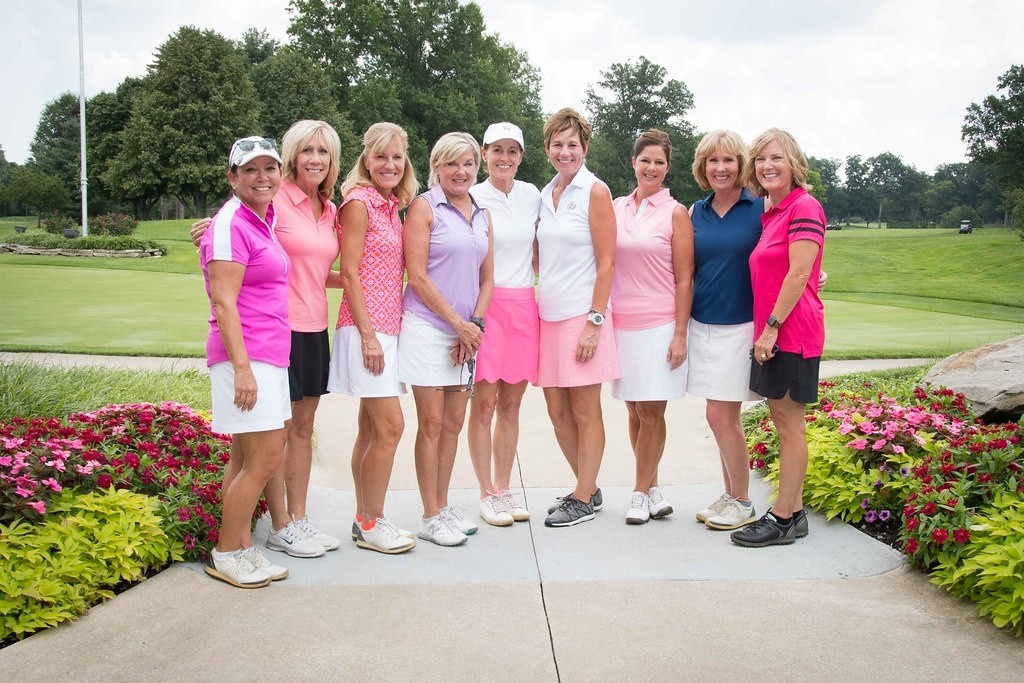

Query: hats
482,122,525,153
228,135,282,169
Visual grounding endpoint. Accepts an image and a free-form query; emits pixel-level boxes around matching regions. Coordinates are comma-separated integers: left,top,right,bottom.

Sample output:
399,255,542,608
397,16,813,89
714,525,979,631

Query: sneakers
792,507,809,537
647,485,673,519
205,550,271,589
266,520,326,558
626,491,651,525
697,494,735,523
242,545,288,582
290,515,341,551
352,511,414,553
497,488,531,522
438,506,478,534
479,491,515,527
705,499,756,530
547,484,602,515
419,511,468,546
545,494,595,527
731,507,795,547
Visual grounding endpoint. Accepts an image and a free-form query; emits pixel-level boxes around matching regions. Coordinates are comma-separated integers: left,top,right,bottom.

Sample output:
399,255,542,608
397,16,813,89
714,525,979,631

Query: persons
192,119,340,561
335,122,416,555
467,123,540,526
538,107,617,528
404,131,493,548
687,132,827,529
729,128,824,547
612,129,694,524
199,135,289,588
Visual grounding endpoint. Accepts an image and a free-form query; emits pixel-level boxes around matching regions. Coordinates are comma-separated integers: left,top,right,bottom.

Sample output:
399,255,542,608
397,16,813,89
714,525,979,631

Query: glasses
230,138,278,165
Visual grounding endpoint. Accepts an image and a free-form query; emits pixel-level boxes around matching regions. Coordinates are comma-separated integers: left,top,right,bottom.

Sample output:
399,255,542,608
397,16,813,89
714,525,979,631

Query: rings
761,354,765,357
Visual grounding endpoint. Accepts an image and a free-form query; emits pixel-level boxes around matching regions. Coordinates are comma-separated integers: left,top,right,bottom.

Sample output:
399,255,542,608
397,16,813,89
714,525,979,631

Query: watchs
767,315,782,328
587,313,604,326
470,317,485,328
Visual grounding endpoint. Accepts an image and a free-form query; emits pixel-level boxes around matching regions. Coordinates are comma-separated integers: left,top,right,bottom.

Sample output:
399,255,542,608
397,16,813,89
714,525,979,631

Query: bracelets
590,308,606,319
